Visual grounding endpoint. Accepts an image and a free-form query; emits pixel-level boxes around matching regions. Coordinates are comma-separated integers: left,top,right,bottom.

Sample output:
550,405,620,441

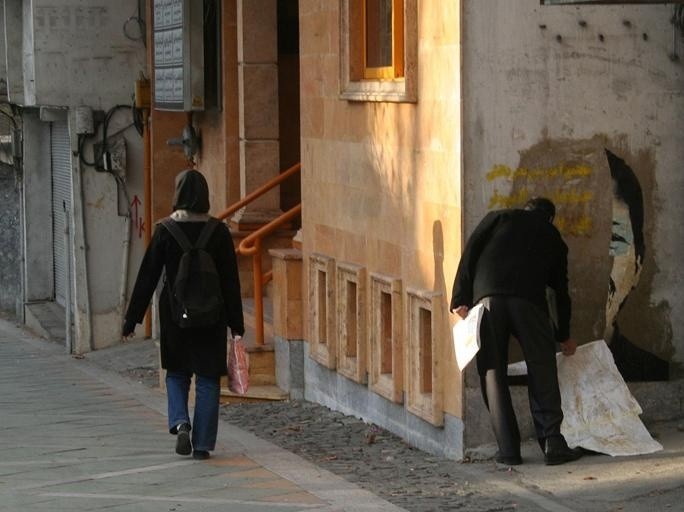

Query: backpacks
159,217,224,330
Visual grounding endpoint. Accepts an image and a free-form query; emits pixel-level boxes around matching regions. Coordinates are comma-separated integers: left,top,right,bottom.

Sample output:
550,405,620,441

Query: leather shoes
495,454,522,465
544,446,584,465
176,423,210,459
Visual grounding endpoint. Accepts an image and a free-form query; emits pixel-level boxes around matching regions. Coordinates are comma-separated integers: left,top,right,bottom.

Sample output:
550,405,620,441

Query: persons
450,197,583,465
121,169,245,459
603,147,669,382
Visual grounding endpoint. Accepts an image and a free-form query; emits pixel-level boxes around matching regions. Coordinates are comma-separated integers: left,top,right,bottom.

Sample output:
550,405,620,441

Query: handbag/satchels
227,336,250,395
